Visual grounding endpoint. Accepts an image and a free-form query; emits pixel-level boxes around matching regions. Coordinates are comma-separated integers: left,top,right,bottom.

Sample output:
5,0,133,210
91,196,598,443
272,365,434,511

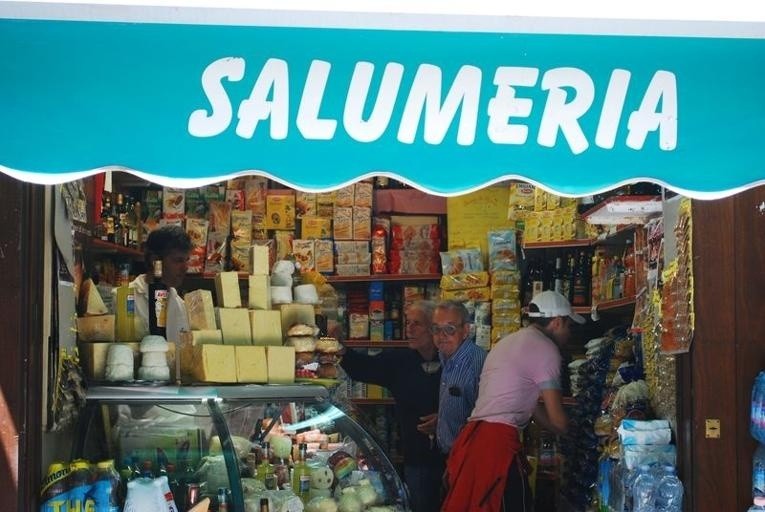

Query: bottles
364,173,412,189
115,263,135,341
747,372,765,512
101,193,142,250
149,260,168,336
391,299,401,341
526,240,636,306
595,447,685,512
40,441,313,512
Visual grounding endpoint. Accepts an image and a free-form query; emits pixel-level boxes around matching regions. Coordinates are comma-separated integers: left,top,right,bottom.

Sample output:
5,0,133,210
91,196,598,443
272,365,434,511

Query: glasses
428,322,464,335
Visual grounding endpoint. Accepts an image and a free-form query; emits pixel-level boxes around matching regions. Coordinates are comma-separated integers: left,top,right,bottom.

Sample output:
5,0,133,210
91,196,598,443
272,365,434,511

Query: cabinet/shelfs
183,272,441,466
70,384,414,511
591,222,644,312
522,238,593,476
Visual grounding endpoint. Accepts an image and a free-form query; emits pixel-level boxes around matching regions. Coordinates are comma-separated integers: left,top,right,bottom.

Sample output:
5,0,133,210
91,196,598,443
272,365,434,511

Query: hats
525,290,586,325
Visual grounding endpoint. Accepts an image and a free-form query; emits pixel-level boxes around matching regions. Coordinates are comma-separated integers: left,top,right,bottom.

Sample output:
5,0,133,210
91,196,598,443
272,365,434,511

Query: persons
440,291,587,512
326,299,443,512
429,302,487,454
115,226,189,332
